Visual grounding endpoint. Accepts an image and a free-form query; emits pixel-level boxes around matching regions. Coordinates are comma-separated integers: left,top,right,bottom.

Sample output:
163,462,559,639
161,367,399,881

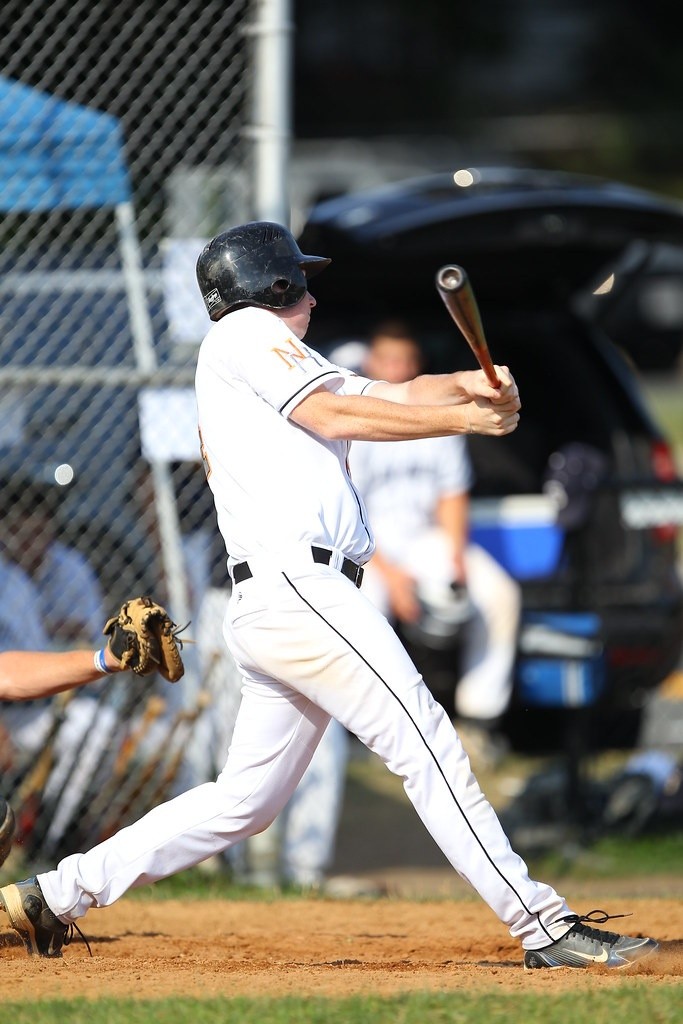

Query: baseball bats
434,263,503,391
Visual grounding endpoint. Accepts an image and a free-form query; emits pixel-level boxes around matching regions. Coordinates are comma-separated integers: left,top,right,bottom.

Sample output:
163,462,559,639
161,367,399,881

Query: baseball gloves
102,595,187,684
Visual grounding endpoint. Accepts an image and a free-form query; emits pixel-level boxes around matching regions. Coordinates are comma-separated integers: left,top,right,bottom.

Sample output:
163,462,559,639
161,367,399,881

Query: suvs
273,165,683,722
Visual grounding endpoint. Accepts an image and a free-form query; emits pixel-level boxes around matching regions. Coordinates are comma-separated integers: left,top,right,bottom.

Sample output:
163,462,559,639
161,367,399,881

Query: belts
234,546,366,588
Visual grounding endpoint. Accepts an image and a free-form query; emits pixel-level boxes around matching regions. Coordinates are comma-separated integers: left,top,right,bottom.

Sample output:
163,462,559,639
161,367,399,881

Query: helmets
197,221,332,322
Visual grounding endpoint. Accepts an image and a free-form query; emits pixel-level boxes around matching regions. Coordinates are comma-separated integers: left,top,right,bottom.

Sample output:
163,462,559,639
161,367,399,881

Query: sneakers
523,921,660,972
0,876,69,960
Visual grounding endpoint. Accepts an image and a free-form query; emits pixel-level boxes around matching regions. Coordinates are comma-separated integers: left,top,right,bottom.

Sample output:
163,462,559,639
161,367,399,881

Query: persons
2,224,657,970
2,598,184,703
4,452,114,877
288,319,520,899
127,445,243,879
